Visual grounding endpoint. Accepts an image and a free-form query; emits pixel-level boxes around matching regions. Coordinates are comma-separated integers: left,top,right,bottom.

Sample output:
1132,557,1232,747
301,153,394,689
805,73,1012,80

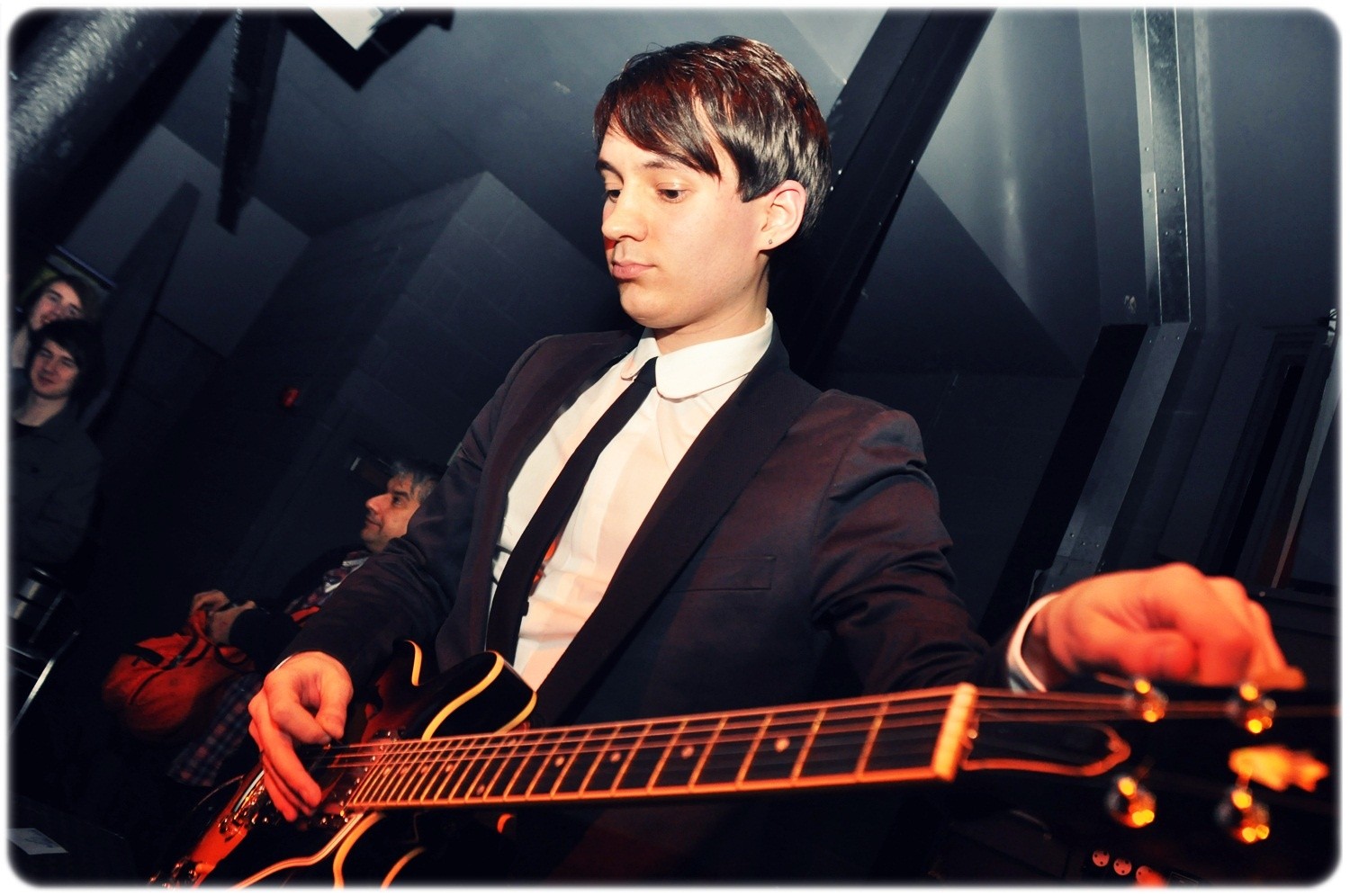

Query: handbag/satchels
103,610,252,734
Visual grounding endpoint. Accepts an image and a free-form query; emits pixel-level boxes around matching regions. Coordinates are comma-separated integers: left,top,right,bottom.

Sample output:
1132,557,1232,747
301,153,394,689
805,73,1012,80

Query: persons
6,266,445,890
249,30,1299,886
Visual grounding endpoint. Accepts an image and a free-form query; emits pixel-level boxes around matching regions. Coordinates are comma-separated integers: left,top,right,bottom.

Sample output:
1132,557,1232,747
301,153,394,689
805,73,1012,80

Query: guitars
141,637,1277,889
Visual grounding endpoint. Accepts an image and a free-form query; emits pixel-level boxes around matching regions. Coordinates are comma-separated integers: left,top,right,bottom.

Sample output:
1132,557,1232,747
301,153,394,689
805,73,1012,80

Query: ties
485,358,656,667
